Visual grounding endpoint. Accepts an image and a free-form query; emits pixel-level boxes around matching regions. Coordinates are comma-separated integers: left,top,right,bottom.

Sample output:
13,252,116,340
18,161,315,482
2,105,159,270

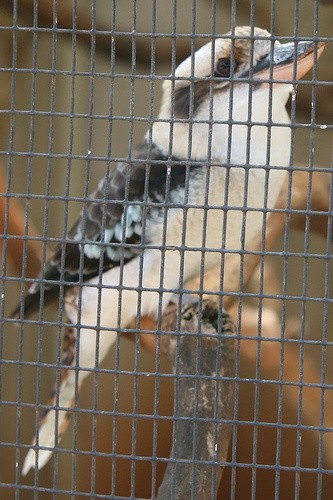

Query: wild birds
8,24,327,474
